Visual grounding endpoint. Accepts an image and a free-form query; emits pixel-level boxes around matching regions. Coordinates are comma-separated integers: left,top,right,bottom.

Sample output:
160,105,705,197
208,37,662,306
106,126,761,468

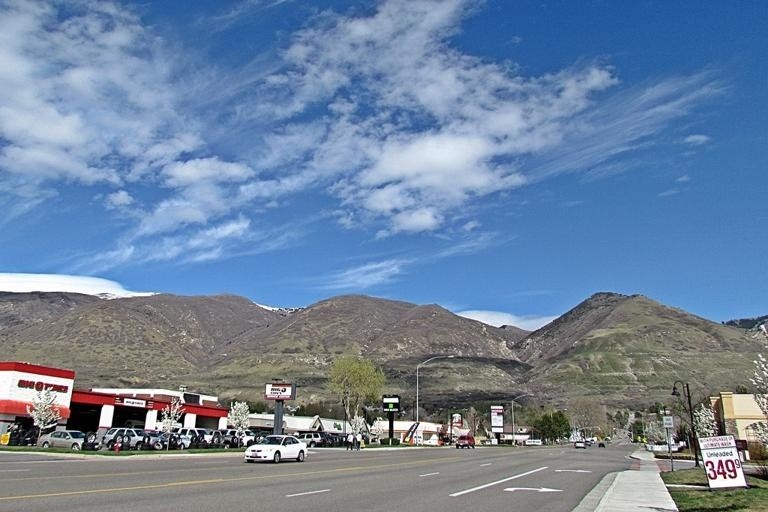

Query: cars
37,430,102,452
244,435,308,463
456,435,475,448
574,438,605,449
101,425,265,452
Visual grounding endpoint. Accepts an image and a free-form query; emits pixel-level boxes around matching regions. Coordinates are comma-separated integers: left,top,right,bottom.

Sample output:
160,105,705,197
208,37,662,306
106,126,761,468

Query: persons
356,432,362,451
347,433,354,451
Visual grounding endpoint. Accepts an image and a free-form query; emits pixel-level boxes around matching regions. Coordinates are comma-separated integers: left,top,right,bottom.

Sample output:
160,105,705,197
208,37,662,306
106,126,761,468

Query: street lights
550,408,567,445
655,402,670,453
510,393,534,445
416,355,454,446
670,380,700,467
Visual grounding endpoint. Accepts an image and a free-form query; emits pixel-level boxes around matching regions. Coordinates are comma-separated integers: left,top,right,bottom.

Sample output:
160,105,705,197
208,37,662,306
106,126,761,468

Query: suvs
296,432,326,448
